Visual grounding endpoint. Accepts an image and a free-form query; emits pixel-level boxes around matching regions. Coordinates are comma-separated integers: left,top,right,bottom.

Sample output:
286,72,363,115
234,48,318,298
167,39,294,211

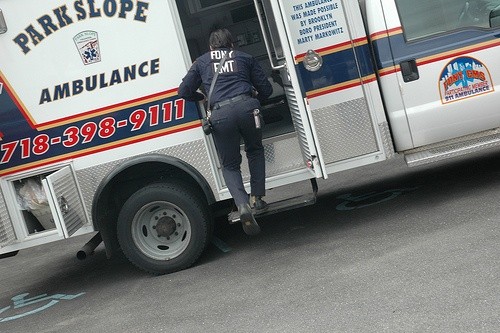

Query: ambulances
1,2,499,279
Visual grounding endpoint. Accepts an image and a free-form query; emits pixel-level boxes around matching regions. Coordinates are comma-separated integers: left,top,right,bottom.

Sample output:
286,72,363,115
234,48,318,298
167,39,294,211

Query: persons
178,28,273,234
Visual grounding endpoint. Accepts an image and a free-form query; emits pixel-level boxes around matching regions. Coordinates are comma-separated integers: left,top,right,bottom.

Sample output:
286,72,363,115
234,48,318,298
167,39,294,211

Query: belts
219,95,242,107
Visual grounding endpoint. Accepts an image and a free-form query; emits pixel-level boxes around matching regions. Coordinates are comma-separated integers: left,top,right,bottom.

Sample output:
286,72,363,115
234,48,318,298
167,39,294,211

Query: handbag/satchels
202,116,212,135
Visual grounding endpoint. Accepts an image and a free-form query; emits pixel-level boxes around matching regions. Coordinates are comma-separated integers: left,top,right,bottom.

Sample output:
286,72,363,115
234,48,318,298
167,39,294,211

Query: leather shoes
238,204,260,236
253,200,268,214
